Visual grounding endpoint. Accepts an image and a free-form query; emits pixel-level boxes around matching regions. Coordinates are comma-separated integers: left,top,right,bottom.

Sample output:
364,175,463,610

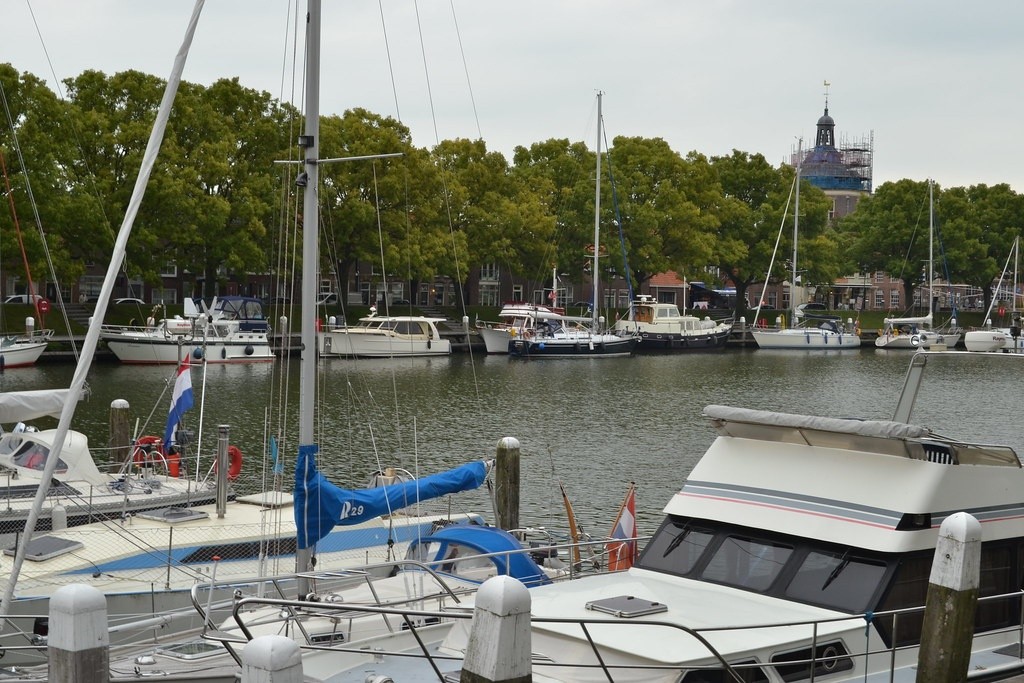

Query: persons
79,291,87,302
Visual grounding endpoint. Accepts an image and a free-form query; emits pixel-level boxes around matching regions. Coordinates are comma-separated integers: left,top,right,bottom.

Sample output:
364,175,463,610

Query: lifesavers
213,446,242,480
523,332,531,339
132,436,166,468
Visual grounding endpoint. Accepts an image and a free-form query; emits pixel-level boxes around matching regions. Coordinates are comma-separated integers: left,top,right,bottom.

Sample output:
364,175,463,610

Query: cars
4,295,42,304
568,302,593,308
751,304,775,310
392,299,409,305
113,298,144,305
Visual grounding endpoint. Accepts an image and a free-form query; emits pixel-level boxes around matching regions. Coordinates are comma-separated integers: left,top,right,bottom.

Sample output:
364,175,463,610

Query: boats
319,304,451,359
0,328,55,370
183,352,1023,683
99,295,276,364
474,261,598,356
613,275,734,349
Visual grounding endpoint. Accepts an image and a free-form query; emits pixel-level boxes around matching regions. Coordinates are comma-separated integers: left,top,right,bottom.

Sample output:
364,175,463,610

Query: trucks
318,293,362,306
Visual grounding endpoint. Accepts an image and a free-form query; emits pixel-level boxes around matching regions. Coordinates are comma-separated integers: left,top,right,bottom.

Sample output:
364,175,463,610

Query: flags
163,353,193,455
607,486,639,570
588,289,593,311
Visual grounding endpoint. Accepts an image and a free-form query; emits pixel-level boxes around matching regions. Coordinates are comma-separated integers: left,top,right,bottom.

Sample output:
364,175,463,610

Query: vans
797,302,826,310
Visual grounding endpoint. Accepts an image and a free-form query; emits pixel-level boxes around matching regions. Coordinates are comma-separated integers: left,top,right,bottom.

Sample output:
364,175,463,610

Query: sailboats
509,91,641,359
0,0,660,682
964,235,1024,355
874,179,962,348
749,139,861,349
